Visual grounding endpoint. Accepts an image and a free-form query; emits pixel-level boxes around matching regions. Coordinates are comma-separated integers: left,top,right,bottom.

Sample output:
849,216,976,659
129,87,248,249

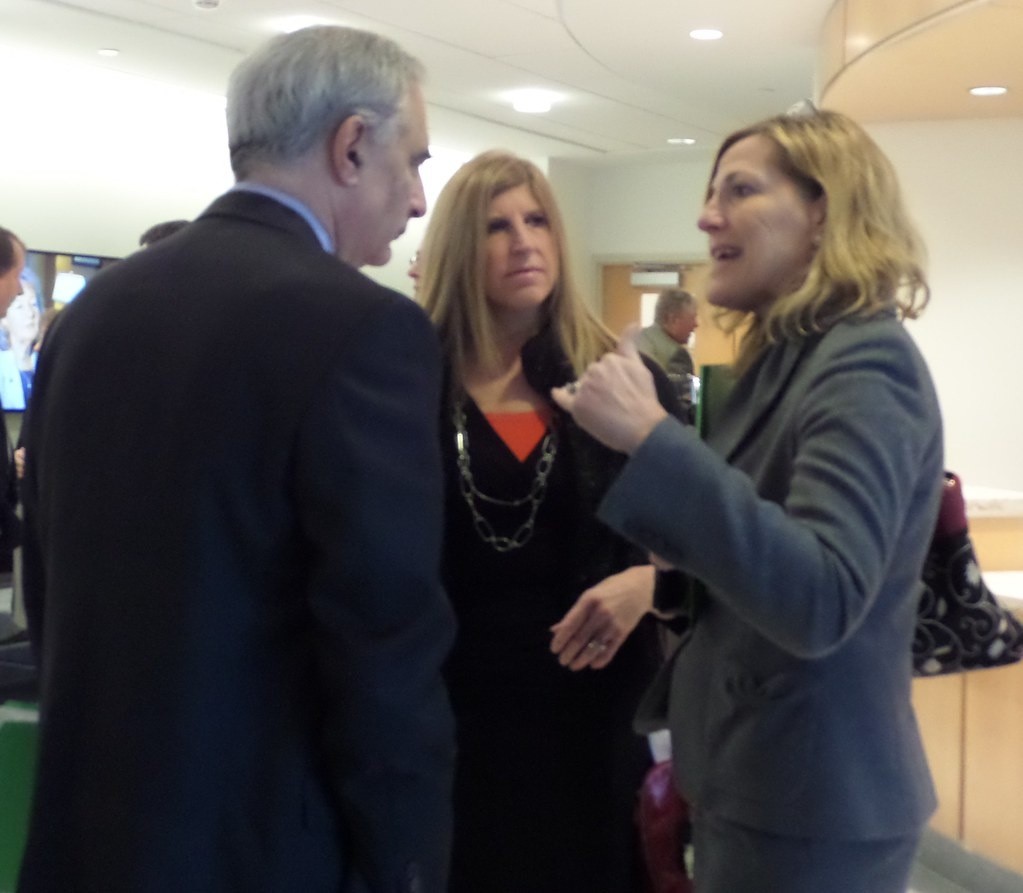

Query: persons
0,220,197,593
416,151,687,893
629,289,698,410
18,24,453,893
551,111,943,893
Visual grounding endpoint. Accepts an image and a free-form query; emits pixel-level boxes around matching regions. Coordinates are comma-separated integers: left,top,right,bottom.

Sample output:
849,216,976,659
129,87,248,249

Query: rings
587,641,606,652
564,382,576,396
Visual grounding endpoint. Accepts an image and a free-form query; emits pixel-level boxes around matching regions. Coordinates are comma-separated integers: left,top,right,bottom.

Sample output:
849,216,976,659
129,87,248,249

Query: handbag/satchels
911,472,1023,677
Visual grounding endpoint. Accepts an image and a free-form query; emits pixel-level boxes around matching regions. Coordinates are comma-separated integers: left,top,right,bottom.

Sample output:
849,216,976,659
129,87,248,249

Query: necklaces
449,394,559,552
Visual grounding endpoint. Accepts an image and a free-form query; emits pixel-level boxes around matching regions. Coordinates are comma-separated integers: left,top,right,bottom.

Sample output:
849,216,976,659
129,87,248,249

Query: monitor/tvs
0,250,122,412
